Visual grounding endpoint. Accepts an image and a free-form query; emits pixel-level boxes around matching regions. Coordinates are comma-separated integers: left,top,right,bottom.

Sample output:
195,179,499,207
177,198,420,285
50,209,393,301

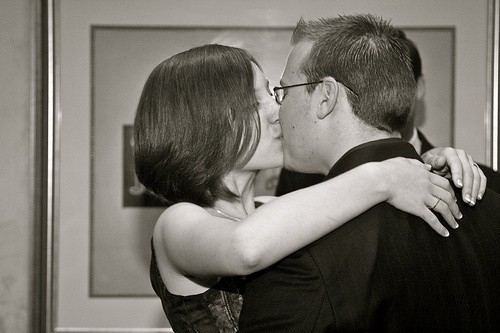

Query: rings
432,199,441,211
470,163,479,168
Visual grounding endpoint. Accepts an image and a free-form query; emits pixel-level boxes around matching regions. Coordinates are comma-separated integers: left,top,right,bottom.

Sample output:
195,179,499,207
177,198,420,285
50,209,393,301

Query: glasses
273,79,360,105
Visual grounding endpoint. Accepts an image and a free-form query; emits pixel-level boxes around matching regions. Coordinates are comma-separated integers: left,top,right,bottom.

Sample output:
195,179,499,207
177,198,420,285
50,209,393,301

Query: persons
272,32,437,198
133,44,487,333
237,15,500,333
